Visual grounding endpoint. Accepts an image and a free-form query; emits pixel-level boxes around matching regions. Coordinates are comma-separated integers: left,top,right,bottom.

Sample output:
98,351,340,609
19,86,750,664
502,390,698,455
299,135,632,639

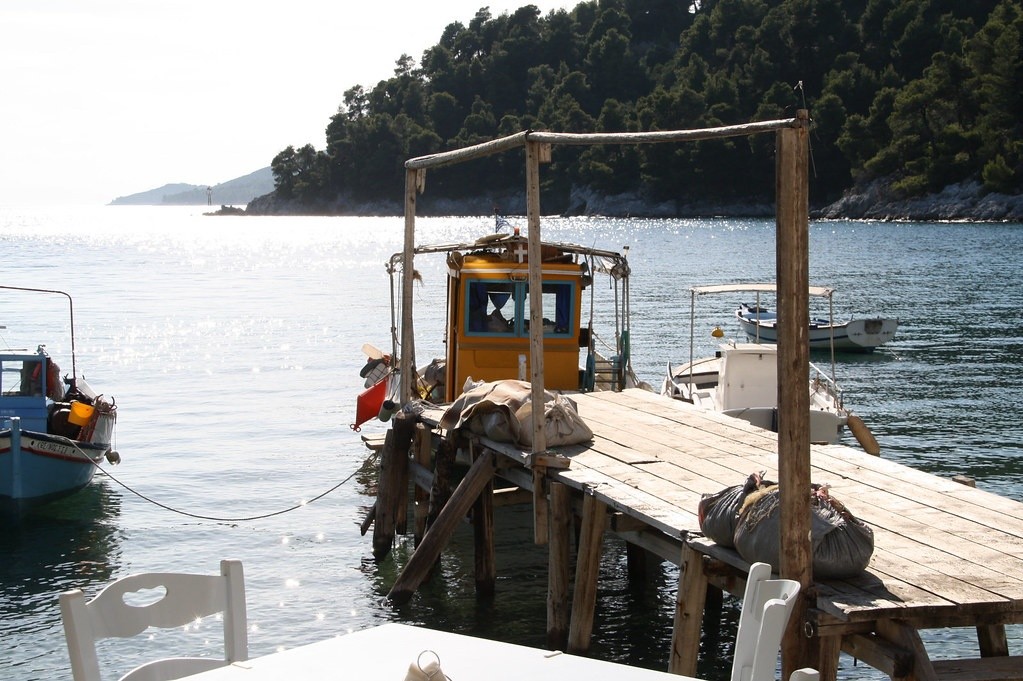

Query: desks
178,623,731,680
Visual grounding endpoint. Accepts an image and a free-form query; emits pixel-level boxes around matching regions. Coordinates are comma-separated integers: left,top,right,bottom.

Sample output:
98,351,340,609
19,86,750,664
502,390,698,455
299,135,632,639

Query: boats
0,286,113,509
661,283,848,444
736,301,901,354
351,225,652,433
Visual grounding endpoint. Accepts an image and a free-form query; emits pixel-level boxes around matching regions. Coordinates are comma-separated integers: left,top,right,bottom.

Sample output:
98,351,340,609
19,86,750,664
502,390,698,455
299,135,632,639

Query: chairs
729,564,801,681
58,559,248,681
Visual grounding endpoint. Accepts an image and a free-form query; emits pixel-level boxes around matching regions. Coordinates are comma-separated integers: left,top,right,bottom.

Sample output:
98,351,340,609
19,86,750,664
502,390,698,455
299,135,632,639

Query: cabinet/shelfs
718,344,777,410
